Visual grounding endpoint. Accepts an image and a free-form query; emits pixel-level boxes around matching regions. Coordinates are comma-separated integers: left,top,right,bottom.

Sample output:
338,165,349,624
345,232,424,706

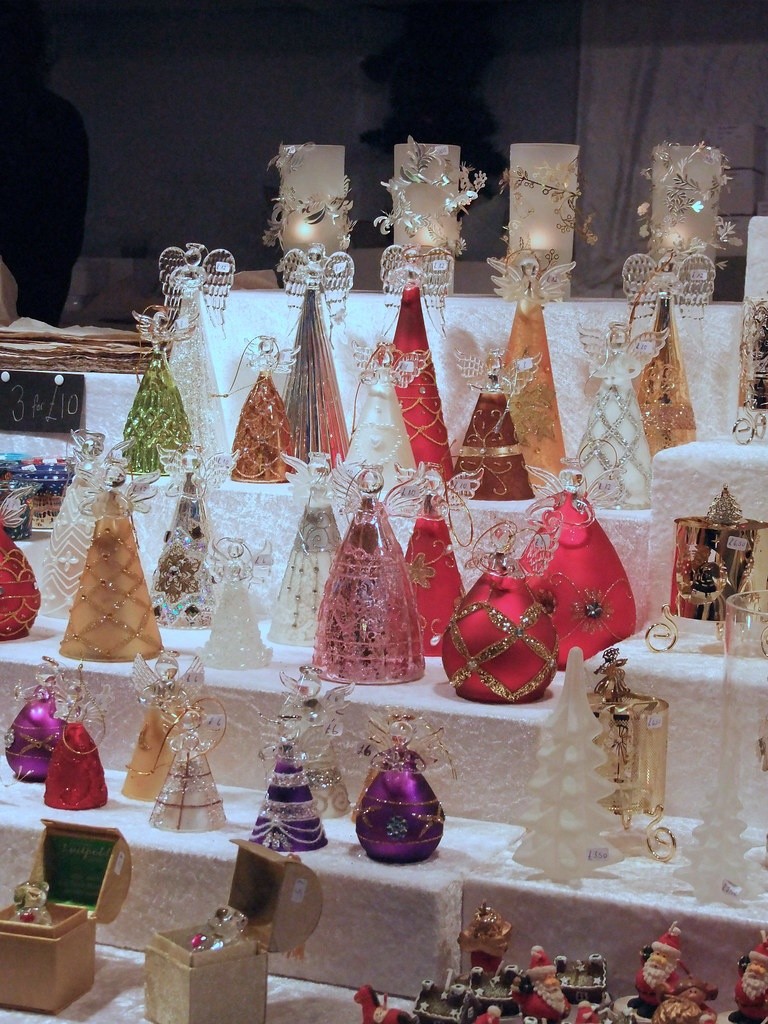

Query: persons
501,946,571,1024
627,921,682,1019
1,1,93,328
575,1001,602,1024
728,930,768,1024
473,1006,501,1024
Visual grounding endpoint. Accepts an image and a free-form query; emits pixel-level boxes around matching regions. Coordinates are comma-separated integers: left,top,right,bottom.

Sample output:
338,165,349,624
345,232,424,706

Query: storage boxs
143,838,324,1024
0,817,132,1014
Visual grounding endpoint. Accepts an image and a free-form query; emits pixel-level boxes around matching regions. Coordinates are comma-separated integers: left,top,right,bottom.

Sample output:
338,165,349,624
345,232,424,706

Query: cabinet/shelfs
1,245,768,1024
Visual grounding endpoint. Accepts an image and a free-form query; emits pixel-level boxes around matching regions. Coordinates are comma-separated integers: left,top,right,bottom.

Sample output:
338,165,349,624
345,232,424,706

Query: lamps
259,143,357,254
375,135,488,295
637,140,744,301
499,143,579,299
